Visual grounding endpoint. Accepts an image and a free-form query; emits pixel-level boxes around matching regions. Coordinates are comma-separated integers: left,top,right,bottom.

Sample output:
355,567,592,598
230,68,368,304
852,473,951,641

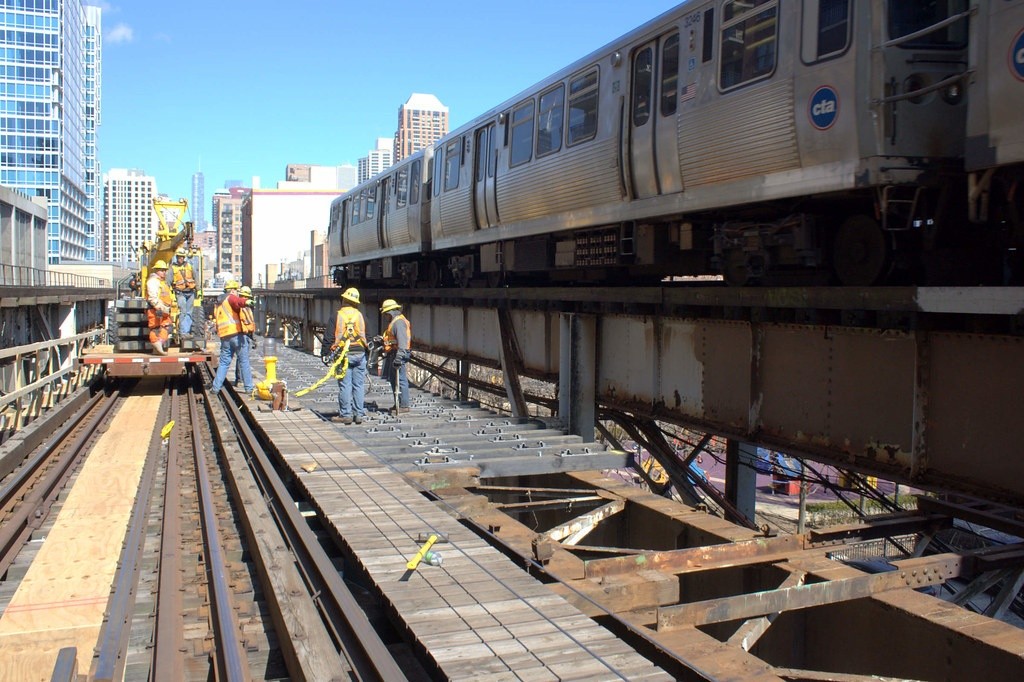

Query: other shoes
331,416,353,423
152,347,160,355
354,417,362,423
150,342,168,356
237,383,255,389
208,392,218,398
391,408,409,413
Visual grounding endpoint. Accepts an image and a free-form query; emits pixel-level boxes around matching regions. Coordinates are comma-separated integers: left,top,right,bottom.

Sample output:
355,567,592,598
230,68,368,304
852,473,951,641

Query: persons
379,300,412,414
144,249,256,394
320,288,368,425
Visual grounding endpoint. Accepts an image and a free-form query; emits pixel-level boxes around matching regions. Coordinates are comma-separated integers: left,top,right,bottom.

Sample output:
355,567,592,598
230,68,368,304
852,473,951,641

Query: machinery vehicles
79,196,213,384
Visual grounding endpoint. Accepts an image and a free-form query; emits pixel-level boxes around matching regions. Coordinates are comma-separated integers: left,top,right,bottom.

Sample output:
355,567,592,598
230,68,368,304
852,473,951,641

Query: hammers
406,530,450,574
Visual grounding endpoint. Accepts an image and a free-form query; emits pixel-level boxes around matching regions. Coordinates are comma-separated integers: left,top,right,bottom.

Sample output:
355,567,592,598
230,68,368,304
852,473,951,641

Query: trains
327,0,1024,333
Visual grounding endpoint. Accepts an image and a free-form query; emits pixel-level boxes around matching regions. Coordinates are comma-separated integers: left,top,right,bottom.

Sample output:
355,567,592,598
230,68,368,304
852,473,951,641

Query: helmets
341,287,360,304
379,299,402,313
224,279,240,289
175,248,187,256
151,260,169,270
238,286,253,297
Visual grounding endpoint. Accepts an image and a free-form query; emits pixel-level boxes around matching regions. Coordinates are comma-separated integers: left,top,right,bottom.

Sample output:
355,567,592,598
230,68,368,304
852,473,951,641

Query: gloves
392,356,403,367
322,356,330,363
